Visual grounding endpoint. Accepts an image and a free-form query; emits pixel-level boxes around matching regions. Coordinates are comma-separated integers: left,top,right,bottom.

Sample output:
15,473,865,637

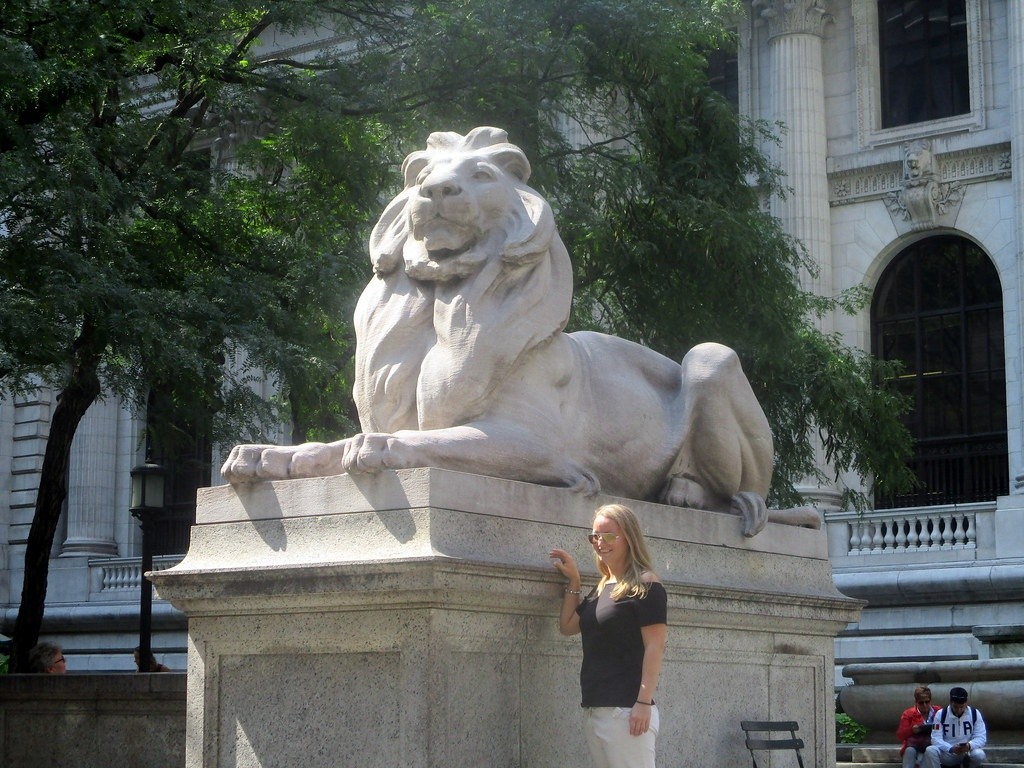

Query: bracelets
565,587,582,594
635,697,652,705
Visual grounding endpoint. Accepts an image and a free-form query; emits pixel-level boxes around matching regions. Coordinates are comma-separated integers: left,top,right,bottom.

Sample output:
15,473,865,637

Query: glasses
588,533,622,545
917,700,931,706
54,656,65,666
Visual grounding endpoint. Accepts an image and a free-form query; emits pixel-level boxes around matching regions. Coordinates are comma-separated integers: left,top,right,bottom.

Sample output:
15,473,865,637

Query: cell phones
959,743,967,746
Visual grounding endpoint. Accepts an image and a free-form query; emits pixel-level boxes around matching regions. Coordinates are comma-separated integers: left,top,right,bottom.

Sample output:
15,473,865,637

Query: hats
951,687,968,702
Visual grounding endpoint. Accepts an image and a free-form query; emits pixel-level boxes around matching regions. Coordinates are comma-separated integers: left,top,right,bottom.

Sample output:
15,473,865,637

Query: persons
550,504,668,768
29,643,66,673
133,646,171,672
925,687,987,768
897,686,943,768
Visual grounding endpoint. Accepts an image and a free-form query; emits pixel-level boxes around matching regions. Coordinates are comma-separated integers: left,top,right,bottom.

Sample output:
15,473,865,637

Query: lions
217,125,822,538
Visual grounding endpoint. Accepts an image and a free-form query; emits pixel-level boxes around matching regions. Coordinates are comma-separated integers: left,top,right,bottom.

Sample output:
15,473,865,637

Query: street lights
125,444,175,677
